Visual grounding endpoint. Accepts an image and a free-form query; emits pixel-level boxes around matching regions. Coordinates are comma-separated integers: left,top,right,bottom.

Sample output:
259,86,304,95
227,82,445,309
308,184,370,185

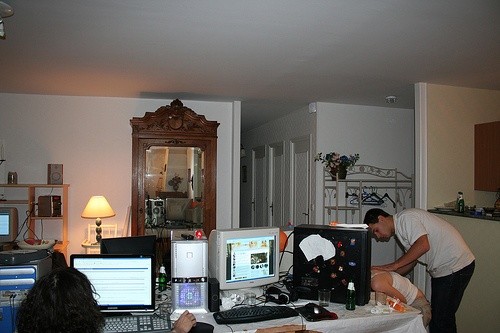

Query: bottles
457,192,464,213
346,279,356,310
159,264,167,292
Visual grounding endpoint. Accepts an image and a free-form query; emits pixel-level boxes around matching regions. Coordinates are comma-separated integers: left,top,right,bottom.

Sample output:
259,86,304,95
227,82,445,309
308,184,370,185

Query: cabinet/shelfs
0,184,71,245
323,163,415,286
474,121,500,192
129,99,221,277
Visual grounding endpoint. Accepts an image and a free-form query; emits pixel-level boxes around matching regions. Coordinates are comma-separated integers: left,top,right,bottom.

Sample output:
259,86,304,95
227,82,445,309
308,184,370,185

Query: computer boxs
170,229,210,321
292,224,373,305
0,248,54,333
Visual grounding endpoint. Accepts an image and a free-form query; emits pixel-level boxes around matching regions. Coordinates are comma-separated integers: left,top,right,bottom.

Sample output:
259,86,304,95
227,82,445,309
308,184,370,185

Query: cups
244,293,256,307
318,289,331,307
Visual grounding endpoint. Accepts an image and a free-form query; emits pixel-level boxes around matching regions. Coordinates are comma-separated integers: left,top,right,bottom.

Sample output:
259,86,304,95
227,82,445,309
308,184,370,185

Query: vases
331,170,347,181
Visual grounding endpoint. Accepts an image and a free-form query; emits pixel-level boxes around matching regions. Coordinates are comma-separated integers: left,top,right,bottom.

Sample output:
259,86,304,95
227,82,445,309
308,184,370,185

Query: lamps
80,195,115,245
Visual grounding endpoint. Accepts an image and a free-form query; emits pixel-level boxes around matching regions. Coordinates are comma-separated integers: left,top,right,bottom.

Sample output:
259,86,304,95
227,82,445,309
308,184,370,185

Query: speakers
207,277,219,312
48,164,63,185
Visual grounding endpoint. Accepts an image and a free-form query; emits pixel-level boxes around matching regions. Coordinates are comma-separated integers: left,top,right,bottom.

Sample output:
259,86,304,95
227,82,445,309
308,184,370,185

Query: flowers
313,148,360,173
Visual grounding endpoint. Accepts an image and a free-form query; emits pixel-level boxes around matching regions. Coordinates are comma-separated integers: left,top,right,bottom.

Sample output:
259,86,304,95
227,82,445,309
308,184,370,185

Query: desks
2,241,70,264
170,281,428,333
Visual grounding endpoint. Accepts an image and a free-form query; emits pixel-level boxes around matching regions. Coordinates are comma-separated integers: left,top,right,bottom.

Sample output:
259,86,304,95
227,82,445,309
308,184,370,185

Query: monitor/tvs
69,254,155,316
207,226,279,303
98,235,156,283
0,207,18,249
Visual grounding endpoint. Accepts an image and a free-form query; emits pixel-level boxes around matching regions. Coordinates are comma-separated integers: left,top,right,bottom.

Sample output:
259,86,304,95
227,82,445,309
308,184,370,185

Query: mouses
310,303,322,314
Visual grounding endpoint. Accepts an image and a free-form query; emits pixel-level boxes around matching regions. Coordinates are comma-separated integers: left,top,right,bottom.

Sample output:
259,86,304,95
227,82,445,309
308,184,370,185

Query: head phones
265,287,292,305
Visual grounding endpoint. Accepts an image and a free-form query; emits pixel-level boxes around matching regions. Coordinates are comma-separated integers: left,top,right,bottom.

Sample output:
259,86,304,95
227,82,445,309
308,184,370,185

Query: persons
363,208,476,333
370,269,432,328
17,268,197,333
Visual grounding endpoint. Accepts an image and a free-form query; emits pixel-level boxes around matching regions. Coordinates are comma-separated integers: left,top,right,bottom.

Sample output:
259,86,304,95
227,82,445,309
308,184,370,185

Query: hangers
348,186,396,208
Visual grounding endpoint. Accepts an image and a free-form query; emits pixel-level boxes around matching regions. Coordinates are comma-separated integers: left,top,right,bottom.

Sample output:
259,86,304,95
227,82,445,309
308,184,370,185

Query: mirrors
145,145,204,275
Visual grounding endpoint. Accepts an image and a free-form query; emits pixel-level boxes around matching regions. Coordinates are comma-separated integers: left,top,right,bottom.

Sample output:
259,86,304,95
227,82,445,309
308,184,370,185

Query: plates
16,239,56,250
434,206,454,211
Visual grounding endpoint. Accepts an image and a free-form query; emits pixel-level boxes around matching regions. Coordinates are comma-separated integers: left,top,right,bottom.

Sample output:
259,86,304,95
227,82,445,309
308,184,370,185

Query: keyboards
99,315,172,333
213,304,299,324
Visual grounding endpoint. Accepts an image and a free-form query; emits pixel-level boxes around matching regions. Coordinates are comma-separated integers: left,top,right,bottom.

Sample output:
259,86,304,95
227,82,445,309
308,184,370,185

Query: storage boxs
473,208,486,216
